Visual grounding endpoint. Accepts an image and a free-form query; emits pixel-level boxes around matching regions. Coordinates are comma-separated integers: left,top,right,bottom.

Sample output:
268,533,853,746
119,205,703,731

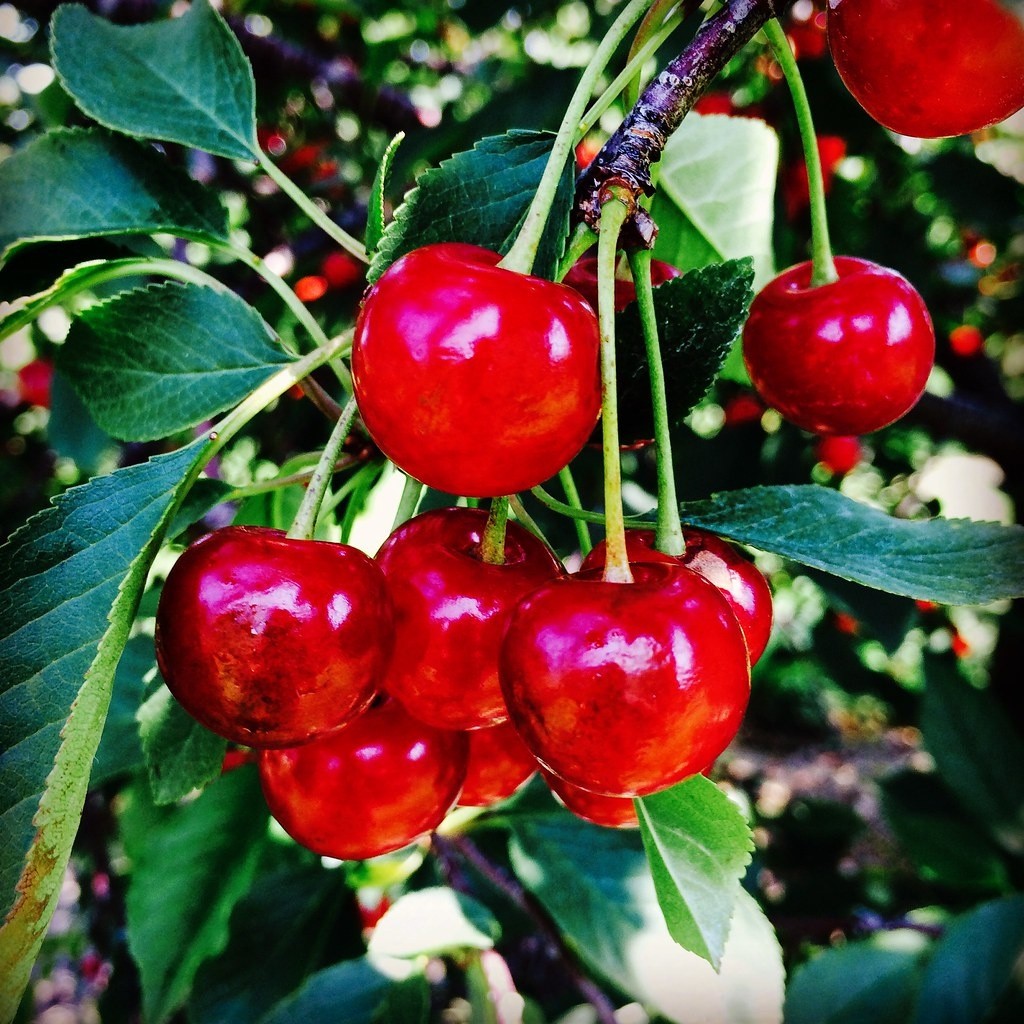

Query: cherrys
152,0,1024,866
